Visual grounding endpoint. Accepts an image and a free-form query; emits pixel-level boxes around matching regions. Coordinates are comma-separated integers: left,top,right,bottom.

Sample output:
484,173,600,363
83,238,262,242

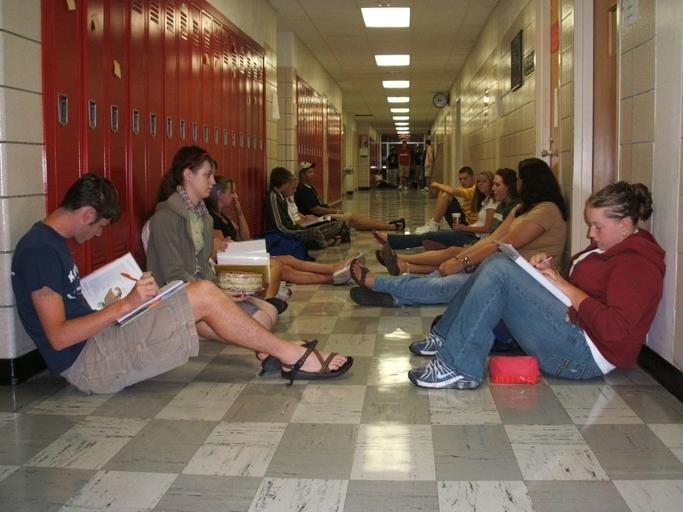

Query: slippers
389,218,405,230
333,243,400,307
373,230,388,245
329,234,344,246
422,239,447,250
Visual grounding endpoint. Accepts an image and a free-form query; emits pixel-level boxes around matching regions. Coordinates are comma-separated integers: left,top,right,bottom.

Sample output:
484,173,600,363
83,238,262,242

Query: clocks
433,93,449,108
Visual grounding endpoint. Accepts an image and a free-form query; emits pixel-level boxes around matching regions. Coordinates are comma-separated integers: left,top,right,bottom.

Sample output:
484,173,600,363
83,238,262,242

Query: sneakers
414,217,439,234
265,285,291,314
398,185,408,191
408,358,479,390
420,186,430,192
409,331,444,355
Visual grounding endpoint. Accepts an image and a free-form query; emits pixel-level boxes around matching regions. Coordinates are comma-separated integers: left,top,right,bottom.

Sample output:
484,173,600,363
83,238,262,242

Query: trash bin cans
343,167,354,195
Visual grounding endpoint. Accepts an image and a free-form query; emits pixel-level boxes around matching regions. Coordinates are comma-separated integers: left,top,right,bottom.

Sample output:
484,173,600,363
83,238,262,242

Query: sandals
281,346,353,380
256,336,318,371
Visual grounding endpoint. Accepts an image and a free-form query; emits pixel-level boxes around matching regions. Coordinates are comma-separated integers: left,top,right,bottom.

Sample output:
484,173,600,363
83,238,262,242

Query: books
117,280,188,327
79,251,143,311
113,279,183,324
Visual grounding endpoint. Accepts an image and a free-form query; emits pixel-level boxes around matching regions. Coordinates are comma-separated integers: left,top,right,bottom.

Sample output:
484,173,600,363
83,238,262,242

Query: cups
451,212,462,229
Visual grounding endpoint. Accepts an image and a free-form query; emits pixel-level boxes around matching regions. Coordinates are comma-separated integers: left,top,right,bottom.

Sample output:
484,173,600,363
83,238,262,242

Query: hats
294,160,315,176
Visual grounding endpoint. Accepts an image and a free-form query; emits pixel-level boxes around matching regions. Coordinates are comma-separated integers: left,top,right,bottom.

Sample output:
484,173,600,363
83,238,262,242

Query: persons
8,172,353,394
368,138,435,191
143,144,279,345
138,160,573,311
404,181,665,392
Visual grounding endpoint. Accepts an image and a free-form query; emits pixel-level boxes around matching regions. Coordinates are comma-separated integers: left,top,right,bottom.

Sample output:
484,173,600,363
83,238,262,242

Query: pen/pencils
120,273,139,281
533,255,556,268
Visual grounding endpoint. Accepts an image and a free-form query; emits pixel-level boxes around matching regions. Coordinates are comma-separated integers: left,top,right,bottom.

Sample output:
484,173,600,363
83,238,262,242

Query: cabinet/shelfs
43,0,270,279
296,74,325,205
326,99,343,205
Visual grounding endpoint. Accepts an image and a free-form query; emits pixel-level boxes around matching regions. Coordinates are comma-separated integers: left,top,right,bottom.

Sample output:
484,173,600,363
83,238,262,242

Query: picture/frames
510,29,523,92
